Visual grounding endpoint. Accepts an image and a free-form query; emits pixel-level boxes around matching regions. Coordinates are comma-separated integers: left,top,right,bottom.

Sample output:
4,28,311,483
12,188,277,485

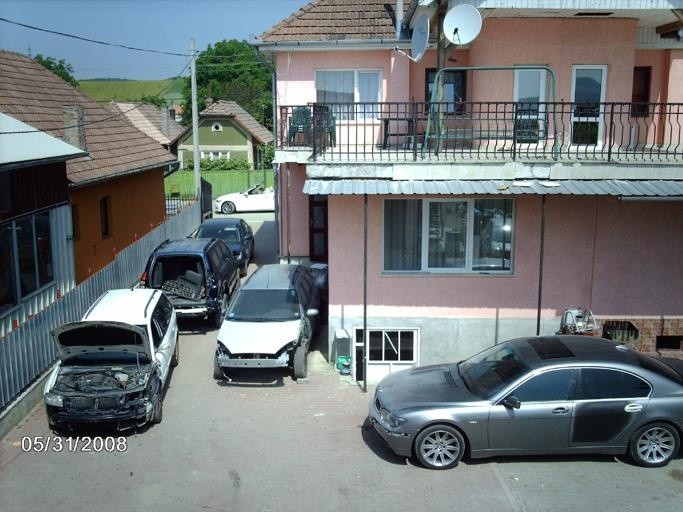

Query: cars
479,218,511,259
368,335,683,470
192,217,255,278
215,184,275,214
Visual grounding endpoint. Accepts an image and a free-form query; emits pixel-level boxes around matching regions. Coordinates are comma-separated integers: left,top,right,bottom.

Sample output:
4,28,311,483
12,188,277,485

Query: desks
378,115,431,151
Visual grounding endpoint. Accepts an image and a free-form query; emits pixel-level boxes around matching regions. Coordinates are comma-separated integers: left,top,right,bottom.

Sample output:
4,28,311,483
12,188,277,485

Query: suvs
140,237,241,330
41,288,180,435
212,264,323,381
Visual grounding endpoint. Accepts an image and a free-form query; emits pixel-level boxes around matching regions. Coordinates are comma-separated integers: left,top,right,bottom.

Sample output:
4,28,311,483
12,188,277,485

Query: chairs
286,107,337,147
